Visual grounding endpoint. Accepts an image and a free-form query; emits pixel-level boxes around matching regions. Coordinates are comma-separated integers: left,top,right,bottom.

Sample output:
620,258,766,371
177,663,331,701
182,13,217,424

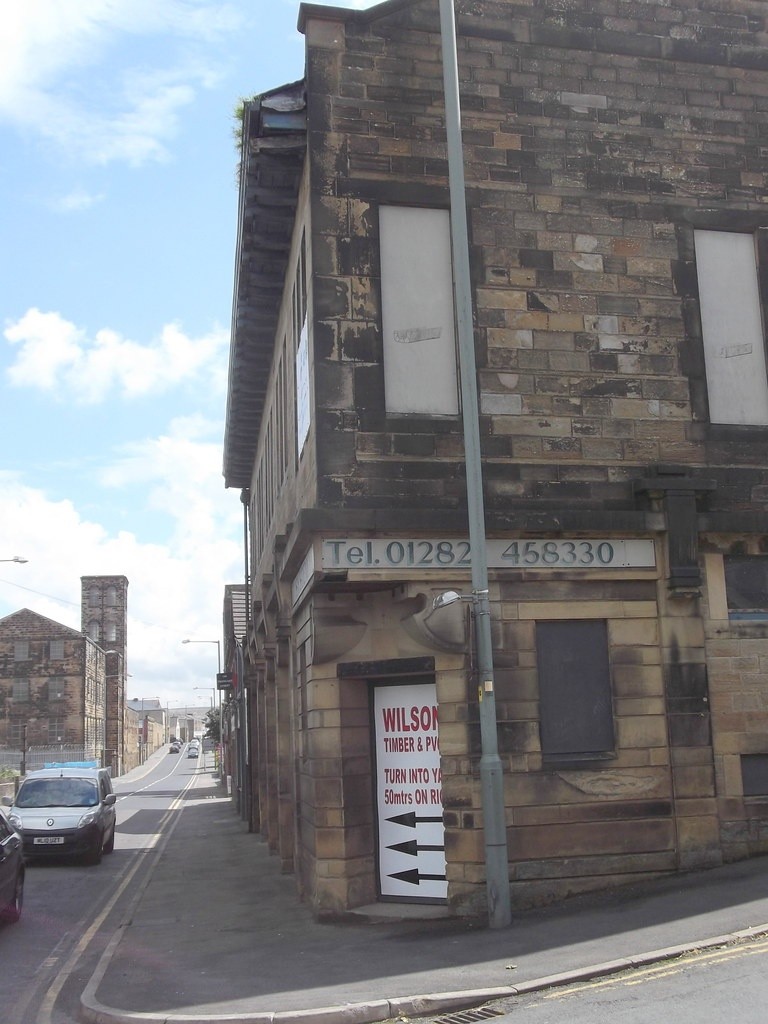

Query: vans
16,766,118,867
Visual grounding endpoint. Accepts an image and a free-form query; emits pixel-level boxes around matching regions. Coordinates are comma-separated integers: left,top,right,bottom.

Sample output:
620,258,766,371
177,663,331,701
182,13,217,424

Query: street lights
0,556,30,565
166,700,179,726
182,638,223,787
192,686,216,749
141,696,159,753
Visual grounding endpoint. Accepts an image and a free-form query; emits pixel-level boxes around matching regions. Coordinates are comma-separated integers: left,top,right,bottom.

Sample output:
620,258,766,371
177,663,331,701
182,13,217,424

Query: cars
187,738,201,749
169,746,180,754
188,749,199,758
173,738,183,749
1,809,25,926
